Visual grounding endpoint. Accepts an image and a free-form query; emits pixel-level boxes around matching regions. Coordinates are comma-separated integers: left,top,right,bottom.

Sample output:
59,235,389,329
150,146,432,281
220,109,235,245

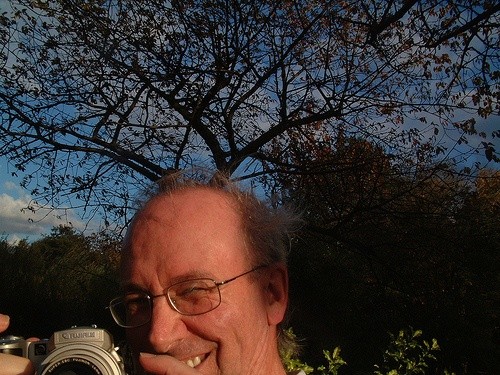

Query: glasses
105,264,268,329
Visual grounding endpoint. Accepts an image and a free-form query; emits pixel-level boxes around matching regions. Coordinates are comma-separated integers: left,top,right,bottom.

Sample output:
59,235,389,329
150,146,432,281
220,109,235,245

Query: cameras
0,324,134,375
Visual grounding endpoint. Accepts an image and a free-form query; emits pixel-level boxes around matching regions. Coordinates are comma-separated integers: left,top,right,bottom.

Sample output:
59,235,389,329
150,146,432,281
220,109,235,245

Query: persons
0,166,307,375
